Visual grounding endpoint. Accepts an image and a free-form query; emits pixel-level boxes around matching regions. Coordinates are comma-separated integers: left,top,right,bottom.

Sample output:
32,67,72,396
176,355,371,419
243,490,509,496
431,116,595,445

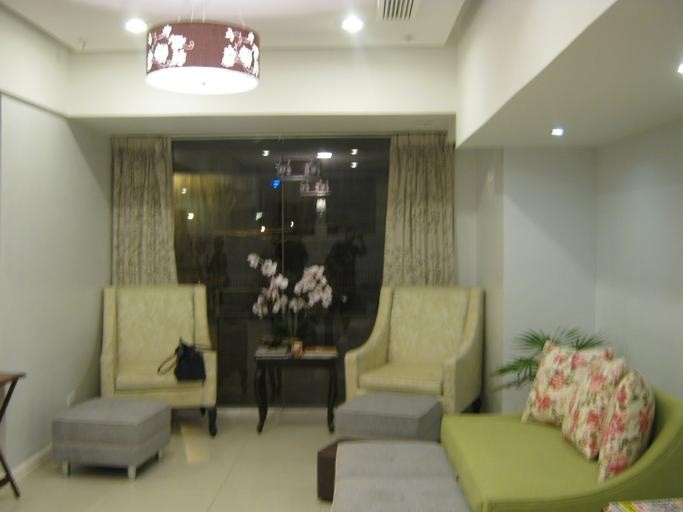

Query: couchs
441,387,683,512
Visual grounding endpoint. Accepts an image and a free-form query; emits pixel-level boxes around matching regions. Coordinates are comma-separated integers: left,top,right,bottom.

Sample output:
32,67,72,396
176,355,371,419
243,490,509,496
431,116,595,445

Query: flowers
242,251,333,341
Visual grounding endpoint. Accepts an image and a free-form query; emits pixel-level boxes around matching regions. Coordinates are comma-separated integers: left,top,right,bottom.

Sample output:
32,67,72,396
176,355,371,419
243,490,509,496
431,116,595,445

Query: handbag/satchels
175,337,205,380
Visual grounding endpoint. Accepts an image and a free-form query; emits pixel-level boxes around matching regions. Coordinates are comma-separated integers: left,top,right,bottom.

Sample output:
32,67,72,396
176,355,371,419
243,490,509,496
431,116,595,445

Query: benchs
323,440,470,512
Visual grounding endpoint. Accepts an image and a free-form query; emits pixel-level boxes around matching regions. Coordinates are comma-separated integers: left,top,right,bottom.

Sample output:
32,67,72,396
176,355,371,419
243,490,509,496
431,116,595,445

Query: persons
270,225,308,321
320,226,366,345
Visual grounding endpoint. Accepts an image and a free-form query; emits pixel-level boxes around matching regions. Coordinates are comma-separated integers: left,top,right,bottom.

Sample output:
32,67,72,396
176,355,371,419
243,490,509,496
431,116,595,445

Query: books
254,341,338,361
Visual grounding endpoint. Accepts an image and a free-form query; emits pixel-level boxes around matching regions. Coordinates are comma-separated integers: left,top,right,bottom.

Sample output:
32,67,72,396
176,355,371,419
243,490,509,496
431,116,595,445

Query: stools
329,388,442,442
51,394,173,480
317,439,338,500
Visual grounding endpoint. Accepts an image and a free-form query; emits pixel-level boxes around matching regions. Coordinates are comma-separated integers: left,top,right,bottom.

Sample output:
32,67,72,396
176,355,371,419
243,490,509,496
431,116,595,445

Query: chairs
345,285,486,418
99,284,220,438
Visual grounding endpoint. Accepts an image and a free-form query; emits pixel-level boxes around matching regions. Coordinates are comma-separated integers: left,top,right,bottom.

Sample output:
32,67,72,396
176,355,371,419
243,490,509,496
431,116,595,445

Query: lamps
145,20,259,96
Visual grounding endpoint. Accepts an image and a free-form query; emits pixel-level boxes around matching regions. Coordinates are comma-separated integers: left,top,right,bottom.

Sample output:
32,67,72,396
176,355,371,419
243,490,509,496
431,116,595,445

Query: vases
287,335,304,359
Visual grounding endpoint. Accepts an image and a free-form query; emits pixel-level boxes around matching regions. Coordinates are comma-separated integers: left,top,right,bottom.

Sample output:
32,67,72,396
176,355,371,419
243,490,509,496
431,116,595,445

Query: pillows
519,342,655,484
